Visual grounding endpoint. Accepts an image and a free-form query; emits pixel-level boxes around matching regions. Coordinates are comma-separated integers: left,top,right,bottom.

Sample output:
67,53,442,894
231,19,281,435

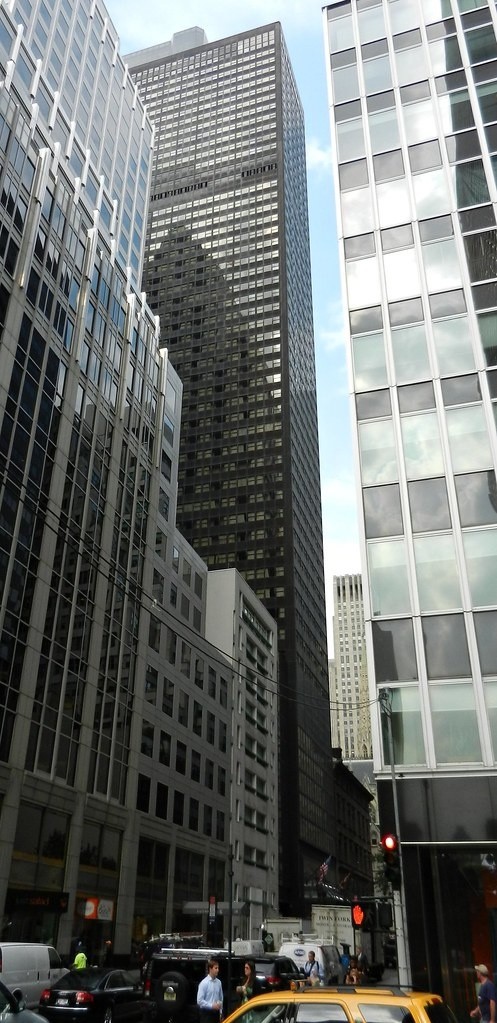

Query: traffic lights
350,901,375,931
382,833,400,883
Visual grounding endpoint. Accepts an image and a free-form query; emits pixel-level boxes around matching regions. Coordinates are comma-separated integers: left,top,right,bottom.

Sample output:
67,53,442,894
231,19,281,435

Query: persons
346,956,361,986
356,945,369,985
470,964,497,1023
71,949,87,970
103,940,114,971
303,951,324,986
197,959,224,1023
236,960,267,1023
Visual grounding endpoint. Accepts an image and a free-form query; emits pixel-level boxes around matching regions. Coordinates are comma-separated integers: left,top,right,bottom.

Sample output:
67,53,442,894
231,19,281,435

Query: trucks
311,904,384,981
264,917,311,955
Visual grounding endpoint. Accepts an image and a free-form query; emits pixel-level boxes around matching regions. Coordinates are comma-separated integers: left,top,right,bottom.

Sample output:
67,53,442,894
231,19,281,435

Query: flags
319,855,331,884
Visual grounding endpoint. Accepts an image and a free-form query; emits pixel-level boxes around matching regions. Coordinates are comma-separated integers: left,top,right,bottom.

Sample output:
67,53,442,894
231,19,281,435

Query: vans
0,942,71,1010
278,932,347,987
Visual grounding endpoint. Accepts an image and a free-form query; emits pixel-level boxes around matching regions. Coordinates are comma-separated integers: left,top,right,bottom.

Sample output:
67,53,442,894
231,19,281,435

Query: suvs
143,948,263,1023
243,954,305,1012
218,984,458,1023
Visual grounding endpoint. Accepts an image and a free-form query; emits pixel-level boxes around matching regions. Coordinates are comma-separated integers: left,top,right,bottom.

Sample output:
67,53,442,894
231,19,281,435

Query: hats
356,944,363,951
475,964,488,976
106,940,112,945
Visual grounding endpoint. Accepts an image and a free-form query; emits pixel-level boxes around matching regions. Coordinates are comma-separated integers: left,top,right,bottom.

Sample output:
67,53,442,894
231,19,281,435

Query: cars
37,966,142,1023
